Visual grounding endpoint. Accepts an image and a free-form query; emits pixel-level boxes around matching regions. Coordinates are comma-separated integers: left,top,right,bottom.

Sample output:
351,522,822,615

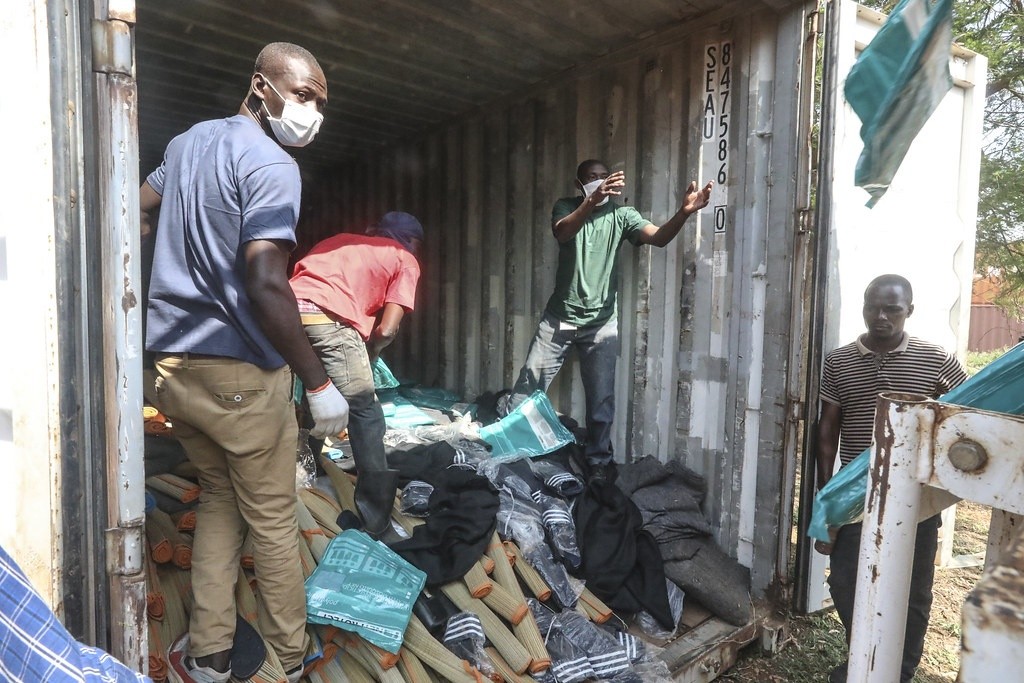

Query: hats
367,209,426,256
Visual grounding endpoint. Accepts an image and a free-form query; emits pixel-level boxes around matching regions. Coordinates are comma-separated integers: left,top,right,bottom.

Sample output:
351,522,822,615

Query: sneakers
168,632,232,683
828,658,847,682
279,651,306,681
588,461,608,482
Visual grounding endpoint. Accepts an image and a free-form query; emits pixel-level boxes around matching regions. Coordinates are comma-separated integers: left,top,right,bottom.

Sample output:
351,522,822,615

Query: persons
815,274,972,683
139,41,350,683
288,211,424,540
502,159,714,479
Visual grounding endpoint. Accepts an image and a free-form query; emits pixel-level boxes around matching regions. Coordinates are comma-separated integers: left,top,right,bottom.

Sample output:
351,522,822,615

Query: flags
843,0,955,210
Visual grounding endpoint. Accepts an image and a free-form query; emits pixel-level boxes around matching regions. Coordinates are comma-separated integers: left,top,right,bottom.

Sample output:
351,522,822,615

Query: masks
573,174,609,210
260,75,323,149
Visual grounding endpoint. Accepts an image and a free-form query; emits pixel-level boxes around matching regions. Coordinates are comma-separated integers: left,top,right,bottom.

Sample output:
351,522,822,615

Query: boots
299,411,329,477
353,469,397,540
